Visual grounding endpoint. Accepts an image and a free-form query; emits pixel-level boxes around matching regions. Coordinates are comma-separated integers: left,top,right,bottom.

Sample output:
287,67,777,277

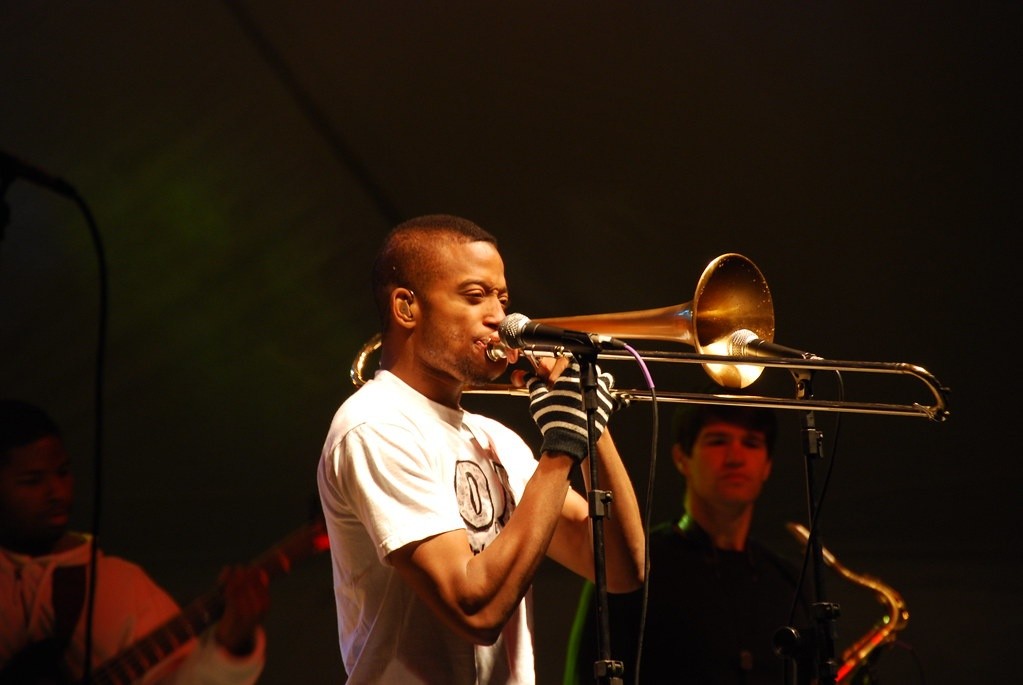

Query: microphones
726,329,825,364
499,314,628,352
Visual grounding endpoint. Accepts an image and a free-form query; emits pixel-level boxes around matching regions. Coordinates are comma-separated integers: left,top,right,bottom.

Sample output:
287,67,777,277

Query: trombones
350,251,952,422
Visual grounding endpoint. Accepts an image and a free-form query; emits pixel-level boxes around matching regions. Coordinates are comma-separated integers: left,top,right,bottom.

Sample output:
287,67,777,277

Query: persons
577,384,834,685
316,214,646,685
0,390,289,685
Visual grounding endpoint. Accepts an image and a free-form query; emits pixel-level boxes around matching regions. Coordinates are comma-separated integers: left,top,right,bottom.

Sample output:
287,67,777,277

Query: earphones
399,300,413,318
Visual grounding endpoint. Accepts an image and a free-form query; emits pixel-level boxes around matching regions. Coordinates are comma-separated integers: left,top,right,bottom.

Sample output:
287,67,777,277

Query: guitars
75,515,329,685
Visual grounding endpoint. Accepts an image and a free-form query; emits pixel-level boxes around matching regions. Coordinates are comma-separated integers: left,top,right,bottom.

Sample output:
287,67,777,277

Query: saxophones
786,518,914,685
